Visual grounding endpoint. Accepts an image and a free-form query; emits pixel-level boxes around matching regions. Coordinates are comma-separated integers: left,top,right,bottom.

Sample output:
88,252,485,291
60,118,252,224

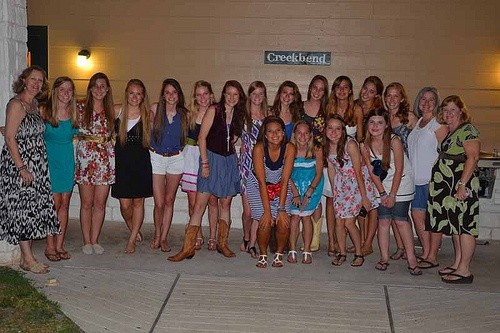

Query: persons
148,77,192,254
267,80,302,254
72,72,118,255
407,86,448,265
359,106,423,277
112,79,154,254
297,74,330,251
322,75,419,261
247,114,297,269
167,78,248,262
0,65,65,273
287,120,325,264
180,81,220,250
323,113,379,268
425,94,481,284
0,76,78,262
238,80,273,251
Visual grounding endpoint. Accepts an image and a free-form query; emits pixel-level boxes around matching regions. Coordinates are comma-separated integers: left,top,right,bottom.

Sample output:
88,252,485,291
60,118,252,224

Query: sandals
19,215,473,284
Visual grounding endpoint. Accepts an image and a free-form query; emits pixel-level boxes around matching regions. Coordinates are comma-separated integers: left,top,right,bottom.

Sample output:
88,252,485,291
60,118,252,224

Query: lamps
76,47,92,66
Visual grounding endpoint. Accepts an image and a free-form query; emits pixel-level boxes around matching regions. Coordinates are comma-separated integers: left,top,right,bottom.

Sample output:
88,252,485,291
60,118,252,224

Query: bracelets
309,185,316,190
293,195,301,198
458,181,467,186
16,165,27,171
200,158,209,169
276,205,286,211
304,194,312,199
